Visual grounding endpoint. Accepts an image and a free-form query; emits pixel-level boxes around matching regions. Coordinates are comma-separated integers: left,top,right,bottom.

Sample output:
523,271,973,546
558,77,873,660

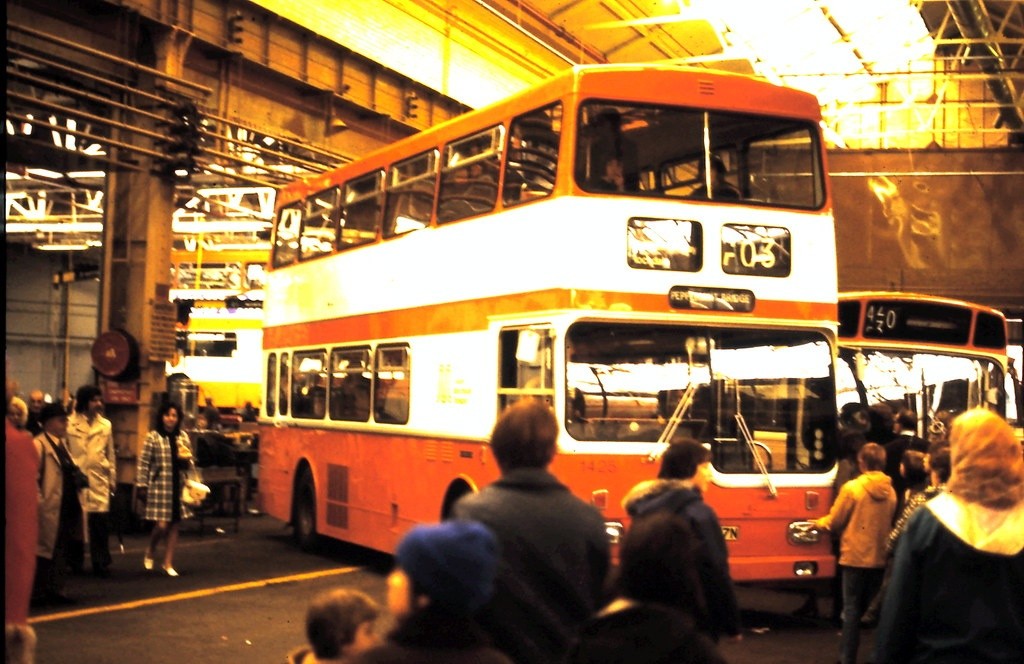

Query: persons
5,379,117,664
807,403,954,664
264,357,722,664
135,402,194,576
621,435,744,646
874,408,1024,664
585,108,741,202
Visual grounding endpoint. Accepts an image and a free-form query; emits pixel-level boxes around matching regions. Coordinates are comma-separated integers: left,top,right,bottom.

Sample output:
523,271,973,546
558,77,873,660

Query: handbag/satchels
181,481,211,507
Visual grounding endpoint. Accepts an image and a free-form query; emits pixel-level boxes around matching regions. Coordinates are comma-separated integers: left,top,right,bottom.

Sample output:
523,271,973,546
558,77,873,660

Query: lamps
327,95,348,136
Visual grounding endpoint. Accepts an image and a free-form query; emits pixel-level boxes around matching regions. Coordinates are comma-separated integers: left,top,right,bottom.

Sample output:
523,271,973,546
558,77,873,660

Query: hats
395,520,500,600
37,403,68,419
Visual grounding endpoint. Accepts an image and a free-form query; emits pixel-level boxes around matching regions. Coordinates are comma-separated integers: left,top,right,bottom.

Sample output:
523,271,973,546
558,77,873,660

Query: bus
168,247,266,415
258,62,837,596
752,290,1023,482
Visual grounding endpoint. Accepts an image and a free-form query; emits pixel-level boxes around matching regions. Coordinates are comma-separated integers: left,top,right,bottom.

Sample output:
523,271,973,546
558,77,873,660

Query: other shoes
144,549,154,570
162,563,179,576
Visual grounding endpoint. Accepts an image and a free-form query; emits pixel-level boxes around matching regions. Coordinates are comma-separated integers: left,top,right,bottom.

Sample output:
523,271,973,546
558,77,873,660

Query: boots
836,622,860,664
